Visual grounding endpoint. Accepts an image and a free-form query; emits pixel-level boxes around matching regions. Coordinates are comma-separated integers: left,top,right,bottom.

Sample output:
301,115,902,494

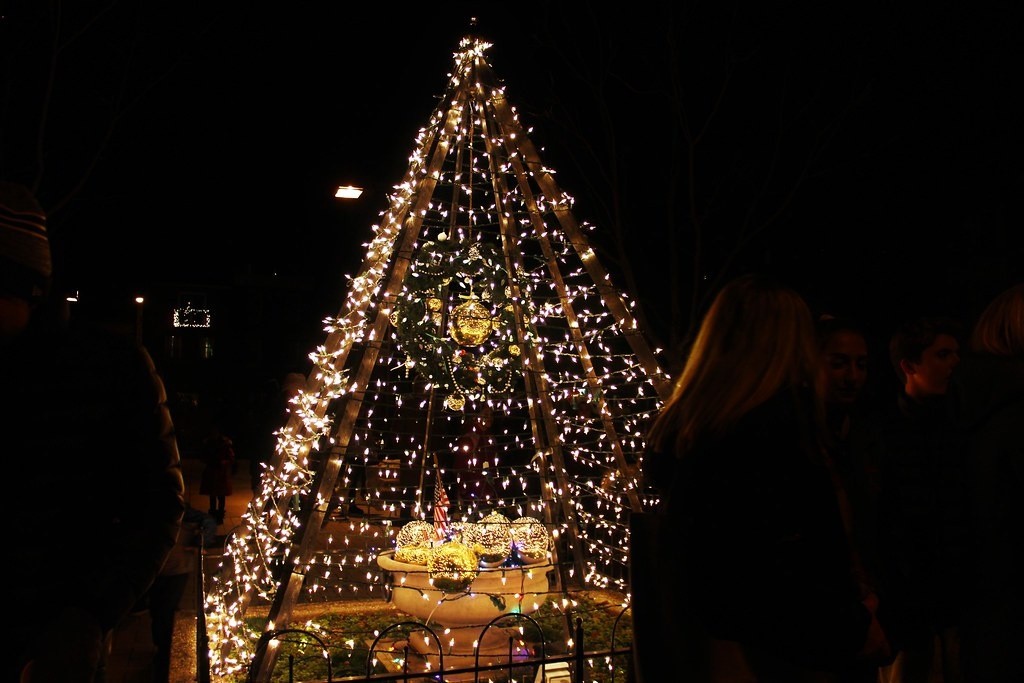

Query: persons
200,427,235,521
0,179,184,683
643,264,1023,683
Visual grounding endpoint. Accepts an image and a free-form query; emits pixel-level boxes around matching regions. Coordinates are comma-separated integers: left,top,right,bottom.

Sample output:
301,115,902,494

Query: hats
0,180,52,276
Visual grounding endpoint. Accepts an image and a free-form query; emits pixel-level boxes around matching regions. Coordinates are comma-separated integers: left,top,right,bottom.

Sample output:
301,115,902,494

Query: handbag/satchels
838,571,896,667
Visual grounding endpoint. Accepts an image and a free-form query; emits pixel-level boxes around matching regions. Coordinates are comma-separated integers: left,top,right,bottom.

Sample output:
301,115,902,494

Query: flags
433,477,450,527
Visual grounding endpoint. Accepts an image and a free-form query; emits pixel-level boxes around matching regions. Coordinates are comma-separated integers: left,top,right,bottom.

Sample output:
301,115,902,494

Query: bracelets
855,575,880,599
